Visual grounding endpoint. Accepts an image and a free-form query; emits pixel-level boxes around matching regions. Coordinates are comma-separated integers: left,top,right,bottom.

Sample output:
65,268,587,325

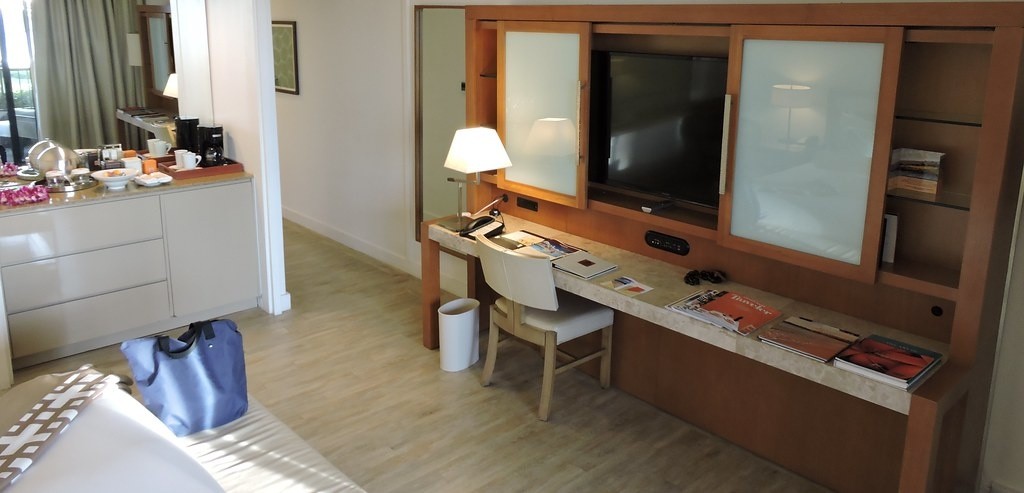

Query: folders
550,251,617,280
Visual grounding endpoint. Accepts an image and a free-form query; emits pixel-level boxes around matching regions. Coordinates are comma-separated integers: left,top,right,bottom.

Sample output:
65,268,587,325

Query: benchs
1,365,367,493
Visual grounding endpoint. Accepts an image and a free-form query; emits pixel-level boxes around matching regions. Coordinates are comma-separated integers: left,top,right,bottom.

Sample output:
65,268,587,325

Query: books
833,334,944,392
551,250,618,280
665,289,781,337
758,315,859,364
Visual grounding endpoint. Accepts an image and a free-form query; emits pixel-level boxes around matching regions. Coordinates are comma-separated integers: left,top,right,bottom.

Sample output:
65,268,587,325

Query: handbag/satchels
119,317,248,439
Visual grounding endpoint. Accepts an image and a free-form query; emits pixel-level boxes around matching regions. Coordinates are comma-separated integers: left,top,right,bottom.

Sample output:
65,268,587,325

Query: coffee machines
196,123,224,168
173,115,199,153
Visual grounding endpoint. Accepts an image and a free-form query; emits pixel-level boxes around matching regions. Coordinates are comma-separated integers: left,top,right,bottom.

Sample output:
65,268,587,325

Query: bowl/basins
91,169,139,190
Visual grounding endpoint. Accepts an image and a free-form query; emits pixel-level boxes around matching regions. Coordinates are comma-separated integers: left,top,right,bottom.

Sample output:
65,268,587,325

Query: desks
420,211,970,493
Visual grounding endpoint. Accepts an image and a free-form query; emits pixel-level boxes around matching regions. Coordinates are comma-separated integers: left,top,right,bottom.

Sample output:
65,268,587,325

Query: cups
174,149,202,170
45,171,65,188
70,167,90,186
146,139,172,157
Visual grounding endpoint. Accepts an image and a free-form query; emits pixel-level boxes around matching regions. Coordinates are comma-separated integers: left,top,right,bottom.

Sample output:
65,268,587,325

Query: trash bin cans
438,298,480,372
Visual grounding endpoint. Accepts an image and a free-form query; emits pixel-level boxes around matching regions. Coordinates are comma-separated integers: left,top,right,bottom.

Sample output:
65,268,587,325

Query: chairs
474,233,614,423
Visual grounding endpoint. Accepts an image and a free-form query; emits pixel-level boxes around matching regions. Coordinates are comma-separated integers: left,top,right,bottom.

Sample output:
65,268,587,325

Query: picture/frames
272,21,300,95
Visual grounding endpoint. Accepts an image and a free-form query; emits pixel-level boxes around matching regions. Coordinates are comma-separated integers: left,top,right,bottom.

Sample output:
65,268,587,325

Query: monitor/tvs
587,49,730,210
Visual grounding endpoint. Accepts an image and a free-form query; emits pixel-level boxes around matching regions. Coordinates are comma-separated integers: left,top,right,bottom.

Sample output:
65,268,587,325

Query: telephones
459,216,504,241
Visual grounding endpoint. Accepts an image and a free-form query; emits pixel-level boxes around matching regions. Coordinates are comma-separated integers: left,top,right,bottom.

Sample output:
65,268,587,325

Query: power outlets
644,230,690,256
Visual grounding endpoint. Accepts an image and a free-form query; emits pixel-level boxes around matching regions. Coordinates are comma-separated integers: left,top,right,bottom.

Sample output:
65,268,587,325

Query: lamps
436,125,511,232
163,73,177,99
